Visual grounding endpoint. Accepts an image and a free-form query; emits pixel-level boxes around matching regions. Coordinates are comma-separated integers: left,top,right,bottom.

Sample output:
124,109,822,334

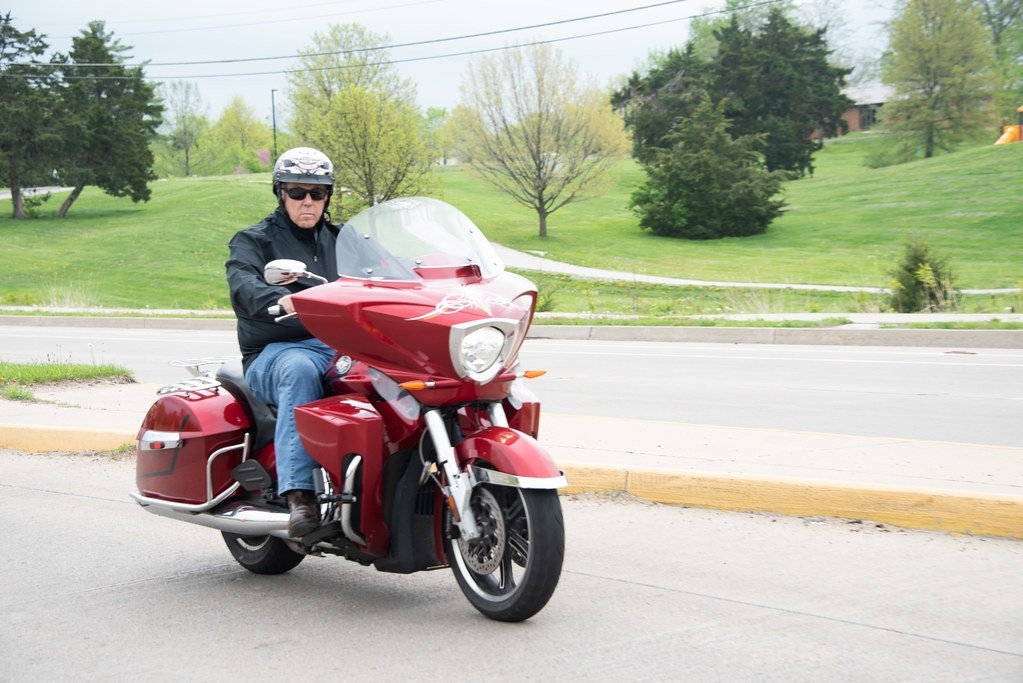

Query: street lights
272,88,279,165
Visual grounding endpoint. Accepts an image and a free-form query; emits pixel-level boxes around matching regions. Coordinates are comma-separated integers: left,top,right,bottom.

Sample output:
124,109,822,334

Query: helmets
272,147,335,195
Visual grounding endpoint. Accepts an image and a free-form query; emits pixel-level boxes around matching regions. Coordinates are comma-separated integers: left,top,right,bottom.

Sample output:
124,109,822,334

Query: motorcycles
125,195,571,625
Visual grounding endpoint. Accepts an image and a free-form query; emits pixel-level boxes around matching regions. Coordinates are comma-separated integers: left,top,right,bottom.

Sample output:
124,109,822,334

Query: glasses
282,188,329,200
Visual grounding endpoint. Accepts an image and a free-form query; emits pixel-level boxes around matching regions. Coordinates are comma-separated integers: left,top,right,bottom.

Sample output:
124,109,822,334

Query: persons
225,147,407,537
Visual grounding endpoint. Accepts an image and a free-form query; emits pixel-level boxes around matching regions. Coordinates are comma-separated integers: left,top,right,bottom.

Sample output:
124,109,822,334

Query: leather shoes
287,489,319,535
